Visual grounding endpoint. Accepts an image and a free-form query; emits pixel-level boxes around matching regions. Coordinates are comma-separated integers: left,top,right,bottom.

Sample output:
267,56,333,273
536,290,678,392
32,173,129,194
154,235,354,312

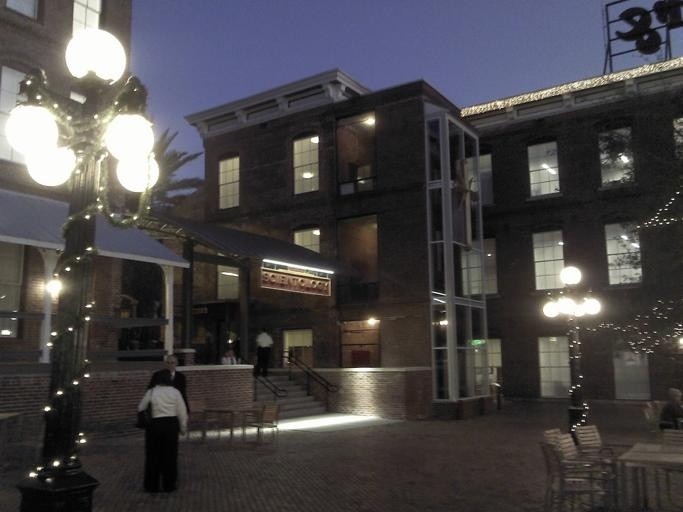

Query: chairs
187,399,281,446
538,400,682,511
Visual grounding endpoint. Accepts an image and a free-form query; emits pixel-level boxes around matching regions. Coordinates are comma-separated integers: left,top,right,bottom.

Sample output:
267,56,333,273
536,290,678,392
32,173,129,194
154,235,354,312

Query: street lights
3,24,164,511
543,266,604,434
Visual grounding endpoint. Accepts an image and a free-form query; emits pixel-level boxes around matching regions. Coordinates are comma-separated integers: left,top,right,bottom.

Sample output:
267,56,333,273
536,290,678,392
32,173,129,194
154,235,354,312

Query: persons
219,348,241,365
658,387,682,429
253,326,274,377
135,369,190,493
145,353,189,422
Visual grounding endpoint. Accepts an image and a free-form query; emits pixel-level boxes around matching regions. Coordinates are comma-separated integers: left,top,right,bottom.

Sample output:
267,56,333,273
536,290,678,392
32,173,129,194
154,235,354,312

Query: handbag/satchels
133,410,150,430
